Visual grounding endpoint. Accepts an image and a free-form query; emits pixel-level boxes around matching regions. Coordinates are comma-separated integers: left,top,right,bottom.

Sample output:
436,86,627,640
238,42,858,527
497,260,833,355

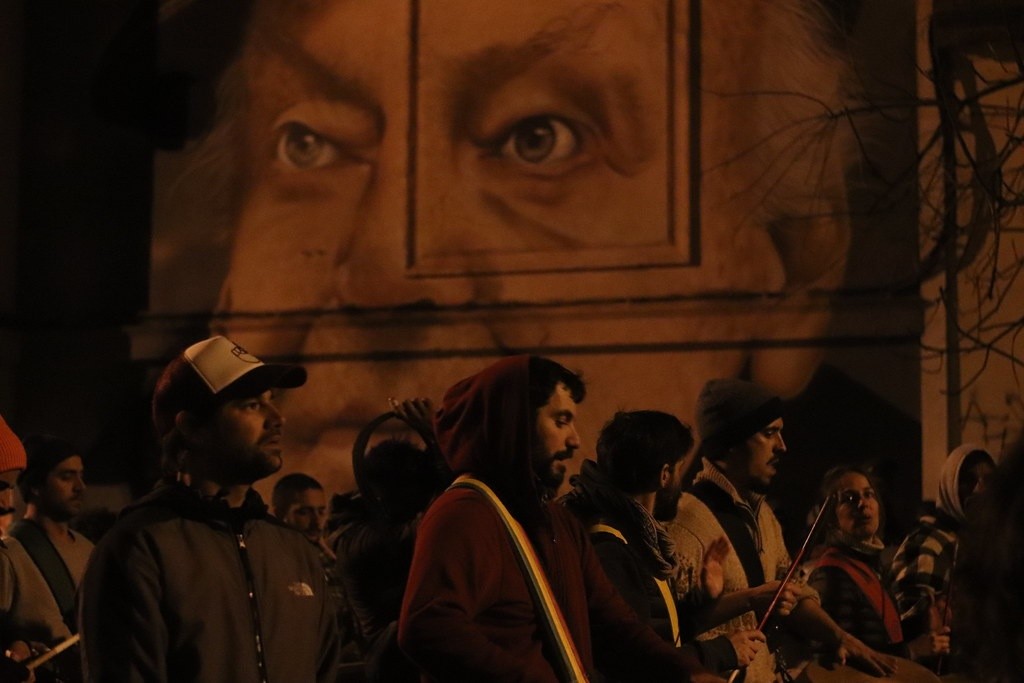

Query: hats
152,336,306,436
15,432,79,490
695,377,783,456
0,415,27,473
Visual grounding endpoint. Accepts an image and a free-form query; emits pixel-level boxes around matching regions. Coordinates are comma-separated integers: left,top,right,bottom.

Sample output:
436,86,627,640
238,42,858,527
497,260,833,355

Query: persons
558,410,766,683
784,464,951,661
0,414,96,683
79,336,368,683
663,378,898,683
322,397,459,683
888,429,1024,683
397,355,726,683
273,471,337,560
